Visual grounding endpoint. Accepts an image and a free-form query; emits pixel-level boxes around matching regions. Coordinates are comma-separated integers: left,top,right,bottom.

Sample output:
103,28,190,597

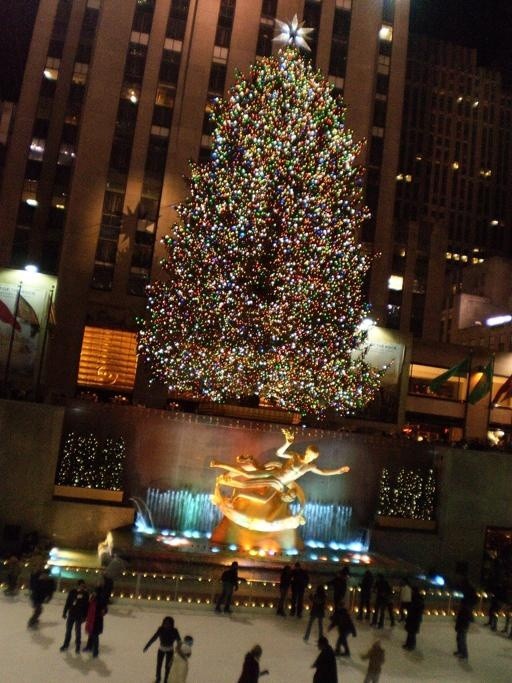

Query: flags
468,355,495,405
430,350,473,391
0,299,22,333
16,295,41,338
46,300,56,342
489,376,512,408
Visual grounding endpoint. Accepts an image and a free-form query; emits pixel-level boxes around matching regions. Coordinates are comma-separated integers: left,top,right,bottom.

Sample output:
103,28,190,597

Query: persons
238,645,269,682
143,616,182,683
166,636,193,683
28,560,45,608
214,562,239,613
27,570,54,626
210,428,351,532
276,562,512,683
60,570,114,658
